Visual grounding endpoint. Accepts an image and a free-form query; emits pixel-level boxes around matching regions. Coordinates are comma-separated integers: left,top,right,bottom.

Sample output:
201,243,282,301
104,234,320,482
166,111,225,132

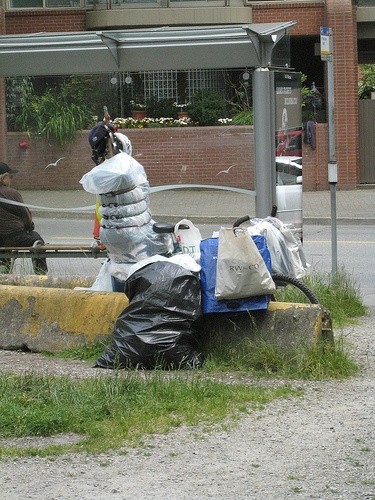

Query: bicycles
152,203,319,305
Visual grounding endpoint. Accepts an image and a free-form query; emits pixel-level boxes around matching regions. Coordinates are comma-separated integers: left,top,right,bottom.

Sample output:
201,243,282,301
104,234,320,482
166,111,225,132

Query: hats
0,161,18,174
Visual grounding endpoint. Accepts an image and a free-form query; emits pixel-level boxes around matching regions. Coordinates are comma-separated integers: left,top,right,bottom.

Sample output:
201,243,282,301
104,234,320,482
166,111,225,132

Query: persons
0,161,48,274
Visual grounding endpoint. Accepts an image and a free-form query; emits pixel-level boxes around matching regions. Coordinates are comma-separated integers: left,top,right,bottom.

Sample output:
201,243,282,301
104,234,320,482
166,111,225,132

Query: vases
177,112,189,119
131,111,147,120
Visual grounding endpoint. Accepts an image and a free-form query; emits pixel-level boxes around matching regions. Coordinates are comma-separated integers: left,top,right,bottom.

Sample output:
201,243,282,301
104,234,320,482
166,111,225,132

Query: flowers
172,101,192,112
130,100,146,110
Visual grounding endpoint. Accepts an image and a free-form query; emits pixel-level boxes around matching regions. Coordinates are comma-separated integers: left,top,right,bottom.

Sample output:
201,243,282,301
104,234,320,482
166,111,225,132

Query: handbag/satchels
168,215,312,313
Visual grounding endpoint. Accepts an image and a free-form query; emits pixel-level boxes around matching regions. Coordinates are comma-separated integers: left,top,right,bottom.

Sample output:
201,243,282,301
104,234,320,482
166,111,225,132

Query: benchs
0,242,107,274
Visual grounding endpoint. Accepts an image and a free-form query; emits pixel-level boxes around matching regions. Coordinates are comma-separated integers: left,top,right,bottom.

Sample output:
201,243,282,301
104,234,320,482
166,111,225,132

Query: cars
275,130,303,242
